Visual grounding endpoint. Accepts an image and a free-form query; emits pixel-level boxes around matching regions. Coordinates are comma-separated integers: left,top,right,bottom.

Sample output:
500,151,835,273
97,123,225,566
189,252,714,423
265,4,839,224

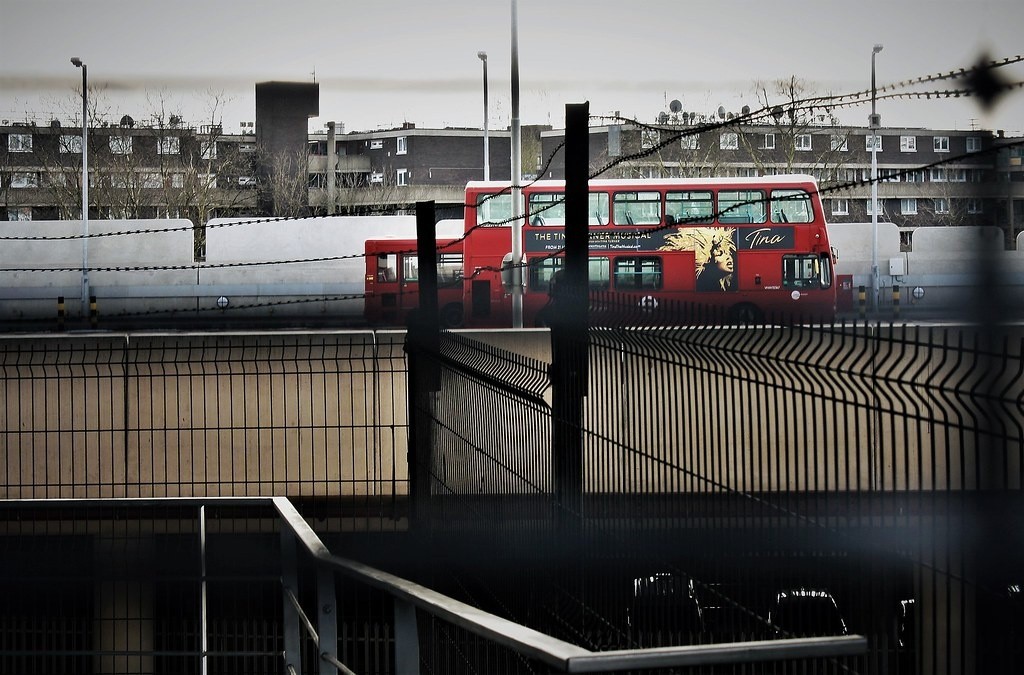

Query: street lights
71,57,92,329
871,43,885,316
479,52,491,181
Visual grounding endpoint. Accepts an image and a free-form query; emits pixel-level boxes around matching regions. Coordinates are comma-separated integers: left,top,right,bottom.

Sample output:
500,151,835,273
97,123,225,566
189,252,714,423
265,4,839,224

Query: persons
658,227,738,293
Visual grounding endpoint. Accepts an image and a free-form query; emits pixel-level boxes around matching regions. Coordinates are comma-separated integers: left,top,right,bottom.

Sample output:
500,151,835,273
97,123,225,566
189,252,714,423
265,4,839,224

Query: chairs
531,208,790,226
381,265,463,283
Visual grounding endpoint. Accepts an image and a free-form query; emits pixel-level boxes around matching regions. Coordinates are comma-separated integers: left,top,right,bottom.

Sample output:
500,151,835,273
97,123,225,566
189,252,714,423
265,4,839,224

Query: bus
464,174,837,329
365,239,463,328
